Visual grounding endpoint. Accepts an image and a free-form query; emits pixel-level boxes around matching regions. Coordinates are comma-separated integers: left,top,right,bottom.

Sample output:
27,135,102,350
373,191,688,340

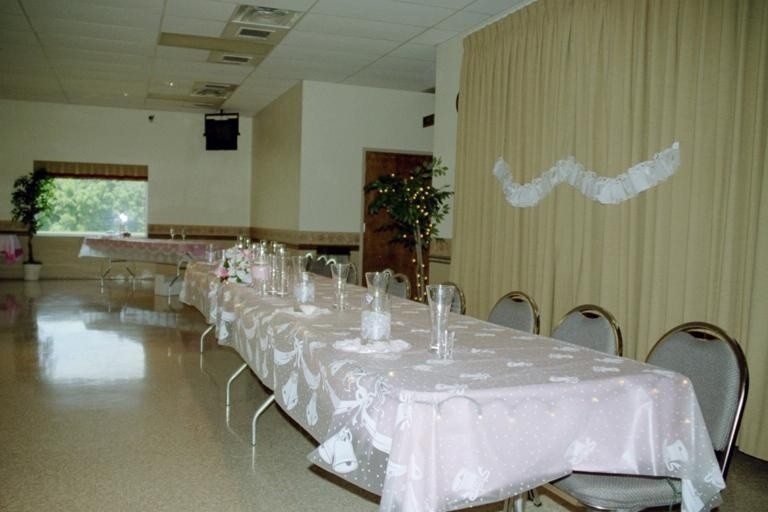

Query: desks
176,247,728,510
84,237,225,304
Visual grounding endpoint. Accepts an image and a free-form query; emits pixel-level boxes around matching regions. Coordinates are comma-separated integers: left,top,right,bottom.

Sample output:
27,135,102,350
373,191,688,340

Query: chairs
377,268,392,292
428,277,466,315
544,321,749,512
301,254,358,285
488,291,540,336
384,275,413,299
501,303,626,510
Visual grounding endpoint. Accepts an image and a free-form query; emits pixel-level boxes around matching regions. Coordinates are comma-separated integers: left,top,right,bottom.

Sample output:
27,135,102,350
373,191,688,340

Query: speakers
206,119,238,149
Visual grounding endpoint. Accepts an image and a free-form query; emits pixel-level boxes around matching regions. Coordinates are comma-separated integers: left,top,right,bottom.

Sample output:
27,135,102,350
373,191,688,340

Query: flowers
213,245,256,286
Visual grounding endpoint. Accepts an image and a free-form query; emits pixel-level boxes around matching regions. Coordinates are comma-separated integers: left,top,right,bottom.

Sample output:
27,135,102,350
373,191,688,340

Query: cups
250,240,456,360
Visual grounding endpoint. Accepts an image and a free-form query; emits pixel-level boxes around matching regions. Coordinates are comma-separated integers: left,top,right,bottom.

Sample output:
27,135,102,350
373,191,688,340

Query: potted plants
10,166,60,282
